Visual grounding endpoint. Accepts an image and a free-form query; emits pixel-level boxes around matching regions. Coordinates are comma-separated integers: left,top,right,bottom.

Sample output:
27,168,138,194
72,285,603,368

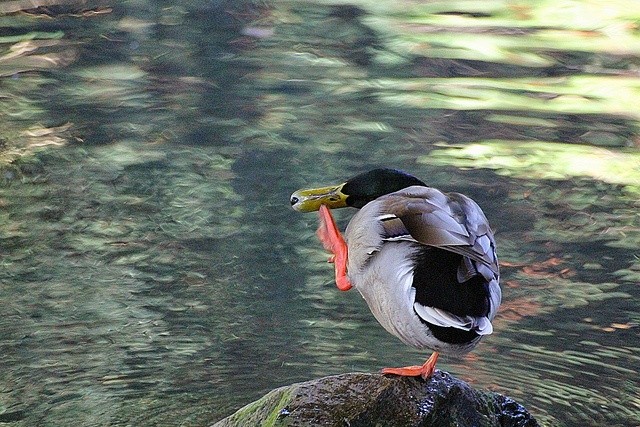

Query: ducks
290,169,501,382
